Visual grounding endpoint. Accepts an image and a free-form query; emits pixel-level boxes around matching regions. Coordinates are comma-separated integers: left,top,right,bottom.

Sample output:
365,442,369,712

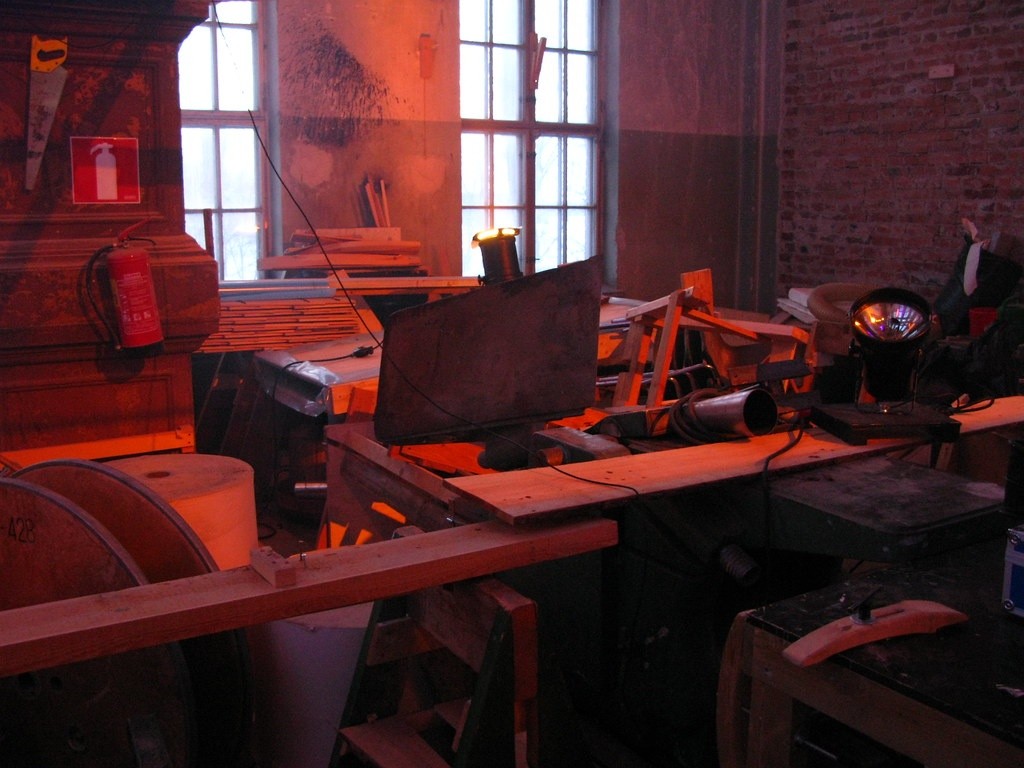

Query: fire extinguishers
84,216,165,352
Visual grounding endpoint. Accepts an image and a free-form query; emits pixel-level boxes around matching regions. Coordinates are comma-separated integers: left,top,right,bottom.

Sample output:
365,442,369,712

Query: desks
744,531,1024,768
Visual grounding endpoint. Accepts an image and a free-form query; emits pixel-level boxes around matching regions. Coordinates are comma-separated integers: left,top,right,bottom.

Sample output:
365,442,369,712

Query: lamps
849,287,935,415
472,226,522,288
